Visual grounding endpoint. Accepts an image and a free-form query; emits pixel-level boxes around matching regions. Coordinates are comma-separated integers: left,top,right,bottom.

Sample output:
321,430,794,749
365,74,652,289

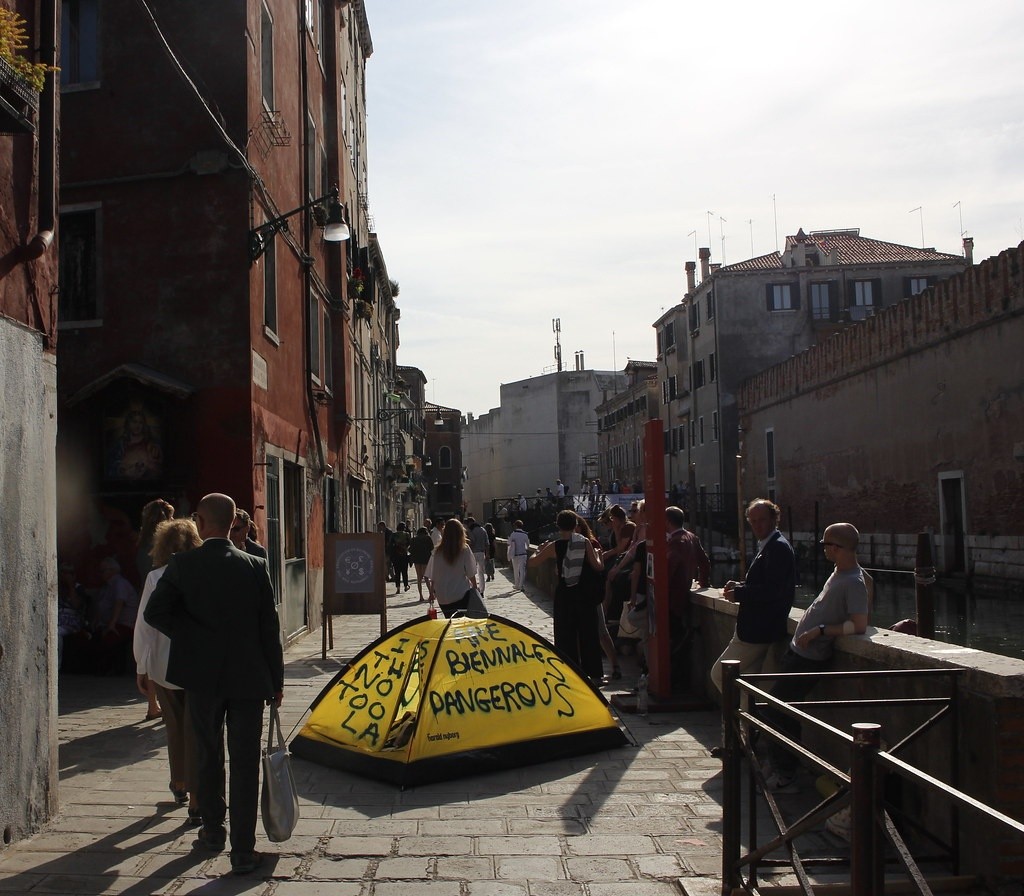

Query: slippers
146,708,161,720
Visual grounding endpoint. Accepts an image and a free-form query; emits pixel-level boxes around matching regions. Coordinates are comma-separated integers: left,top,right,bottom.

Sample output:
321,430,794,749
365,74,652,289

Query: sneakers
198,828,226,851
229,849,261,874
756,770,801,797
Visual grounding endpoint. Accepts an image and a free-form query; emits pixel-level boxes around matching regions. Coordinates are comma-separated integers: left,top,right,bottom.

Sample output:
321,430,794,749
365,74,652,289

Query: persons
581,480,590,494
143,493,284,873
756,523,873,794
132,519,203,824
535,488,542,510
58,568,83,671
518,493,528,511
423,519,477,619
463,517,495,598
555,479,564,510
495,504,508,519
591,480,602,494
608,479,632,494
423,519,445,593
391,522,410,594
405,521,411,538
376,522,394,582
409,528,434,601
506,519,530,590
546,487,555,510
134,499,175,720
710,498,795,755
526,510,622,686
93,559,140,676
599,506,710,684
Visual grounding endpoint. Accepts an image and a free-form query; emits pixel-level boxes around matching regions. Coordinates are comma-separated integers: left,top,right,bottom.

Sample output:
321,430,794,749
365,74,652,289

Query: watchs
819,623,824,635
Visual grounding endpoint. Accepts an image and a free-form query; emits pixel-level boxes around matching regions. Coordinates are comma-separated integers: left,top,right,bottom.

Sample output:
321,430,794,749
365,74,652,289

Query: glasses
819,540,844,549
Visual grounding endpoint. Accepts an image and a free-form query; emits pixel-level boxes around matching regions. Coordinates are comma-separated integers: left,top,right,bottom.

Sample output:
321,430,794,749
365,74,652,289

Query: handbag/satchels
263,703,299,842
507,541,515,561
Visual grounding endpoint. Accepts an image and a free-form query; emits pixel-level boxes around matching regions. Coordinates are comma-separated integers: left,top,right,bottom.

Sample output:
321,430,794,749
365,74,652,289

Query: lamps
425,457,432,465
434,411,444,425
323,192,350,241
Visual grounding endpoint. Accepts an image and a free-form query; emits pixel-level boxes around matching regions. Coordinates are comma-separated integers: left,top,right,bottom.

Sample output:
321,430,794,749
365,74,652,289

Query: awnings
387,393,400,402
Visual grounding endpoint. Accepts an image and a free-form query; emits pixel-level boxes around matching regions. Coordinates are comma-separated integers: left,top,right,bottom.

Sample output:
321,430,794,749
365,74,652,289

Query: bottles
636,674,647,716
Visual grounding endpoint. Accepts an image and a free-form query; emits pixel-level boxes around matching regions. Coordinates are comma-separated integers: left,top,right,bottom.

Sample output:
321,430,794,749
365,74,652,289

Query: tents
289,609,640,791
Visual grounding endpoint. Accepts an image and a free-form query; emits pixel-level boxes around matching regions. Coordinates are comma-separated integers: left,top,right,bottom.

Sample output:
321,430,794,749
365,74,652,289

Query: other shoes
186,812,202,827
592,676,604,685
486,575,494,580
481,592,485,597
711,746,726,756
404,586,409,590
396,589,401,594
611,666,625,680
168,780,188,805
512,584,525,591
419,597,435,602
